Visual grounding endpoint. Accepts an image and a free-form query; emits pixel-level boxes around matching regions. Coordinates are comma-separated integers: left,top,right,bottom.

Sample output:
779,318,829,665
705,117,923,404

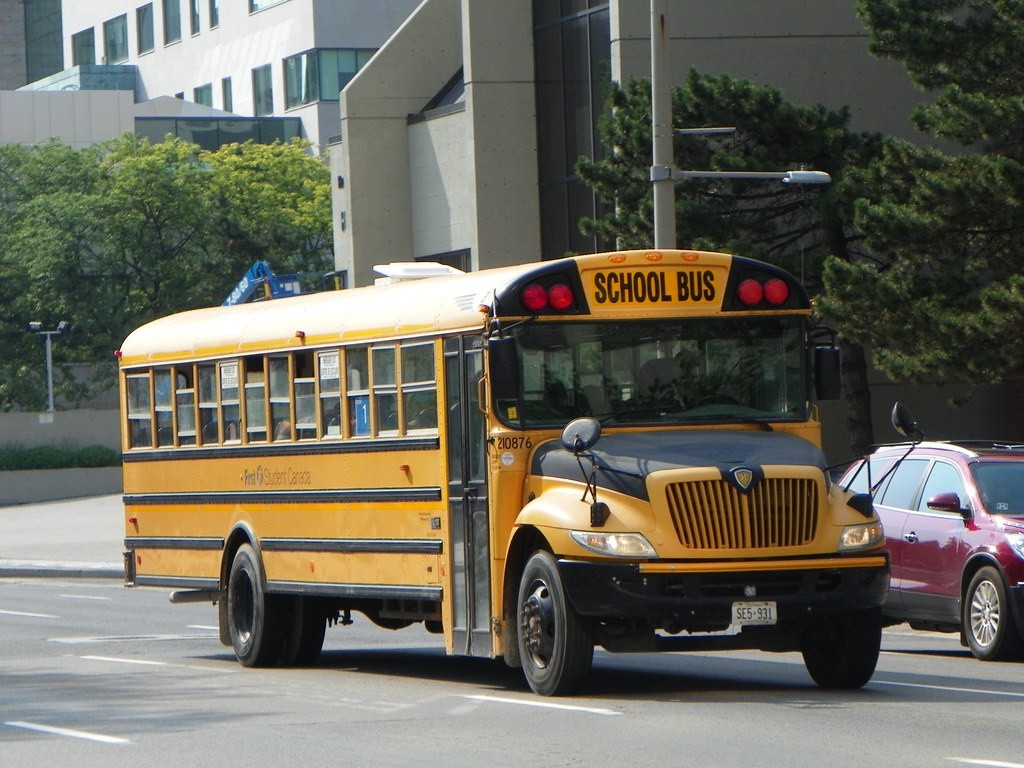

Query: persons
518,342,783,421
139,389,438,447
449,370,486,481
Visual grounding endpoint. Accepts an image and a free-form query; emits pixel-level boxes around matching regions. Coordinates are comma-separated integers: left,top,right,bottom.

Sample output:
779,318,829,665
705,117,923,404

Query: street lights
28,318,72,414
653,164,832,251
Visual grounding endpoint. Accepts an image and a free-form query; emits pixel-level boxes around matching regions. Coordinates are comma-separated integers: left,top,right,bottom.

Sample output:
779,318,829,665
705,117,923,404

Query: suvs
829,435,1024,663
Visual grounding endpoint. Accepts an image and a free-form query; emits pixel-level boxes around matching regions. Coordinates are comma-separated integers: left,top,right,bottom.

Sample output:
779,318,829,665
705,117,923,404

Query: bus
114,246,925,699
